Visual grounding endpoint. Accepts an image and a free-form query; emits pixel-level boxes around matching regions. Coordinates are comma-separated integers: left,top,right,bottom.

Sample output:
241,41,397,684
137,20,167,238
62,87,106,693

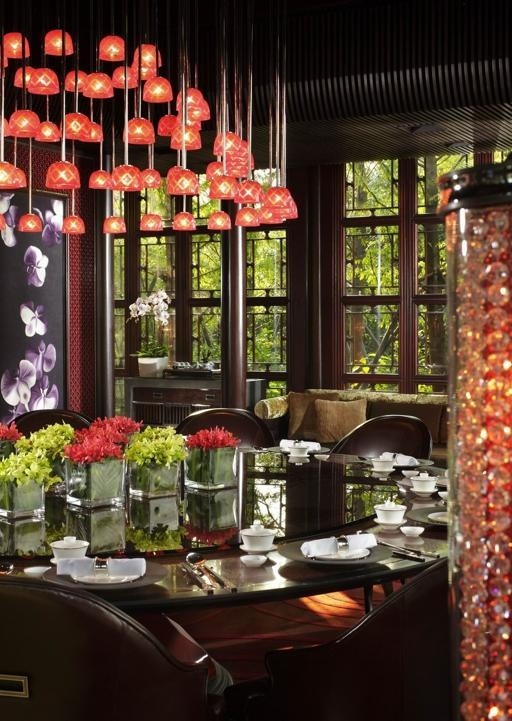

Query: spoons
357,530,410,553
181,553,237,593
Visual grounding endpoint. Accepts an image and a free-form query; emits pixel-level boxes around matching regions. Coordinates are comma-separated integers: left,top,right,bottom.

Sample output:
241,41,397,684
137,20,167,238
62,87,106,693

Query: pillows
312,399,367,441
286,391,339,441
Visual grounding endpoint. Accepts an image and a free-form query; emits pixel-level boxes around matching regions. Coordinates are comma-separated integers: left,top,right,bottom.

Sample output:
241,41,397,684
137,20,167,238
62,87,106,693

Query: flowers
124,288,175,357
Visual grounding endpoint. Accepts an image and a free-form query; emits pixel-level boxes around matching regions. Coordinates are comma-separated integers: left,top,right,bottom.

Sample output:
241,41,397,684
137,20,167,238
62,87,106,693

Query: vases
137,356,168,378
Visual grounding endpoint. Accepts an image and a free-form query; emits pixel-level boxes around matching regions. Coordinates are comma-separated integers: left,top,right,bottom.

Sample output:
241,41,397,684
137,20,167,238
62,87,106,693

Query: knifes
334,531,366,556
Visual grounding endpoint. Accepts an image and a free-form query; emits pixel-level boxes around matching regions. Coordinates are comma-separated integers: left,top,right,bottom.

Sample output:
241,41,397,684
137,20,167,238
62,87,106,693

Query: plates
23,566,47,577
370,459,438,497
363,458,434,470
174,361,190,368
240,544,278,567
406,492,448,526
373,518,425,537
315,545,370,561
49,557,142,583
287,457,310,463
268,446,330,454
287,454,310,457
313,455,331,461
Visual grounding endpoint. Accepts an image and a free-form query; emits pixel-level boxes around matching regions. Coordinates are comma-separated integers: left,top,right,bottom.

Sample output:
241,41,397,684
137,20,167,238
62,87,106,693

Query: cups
374,502,407,522
240,524,276,550
50,536,90,560
378,453,416,469
280,438,321,455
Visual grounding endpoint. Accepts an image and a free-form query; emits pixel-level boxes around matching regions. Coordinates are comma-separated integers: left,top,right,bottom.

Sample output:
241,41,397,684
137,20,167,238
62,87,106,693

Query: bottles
194,360,200,370
203,362,213,370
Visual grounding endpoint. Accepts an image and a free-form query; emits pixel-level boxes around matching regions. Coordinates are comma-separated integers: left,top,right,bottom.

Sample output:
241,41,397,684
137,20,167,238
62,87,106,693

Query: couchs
253,387,449,468
